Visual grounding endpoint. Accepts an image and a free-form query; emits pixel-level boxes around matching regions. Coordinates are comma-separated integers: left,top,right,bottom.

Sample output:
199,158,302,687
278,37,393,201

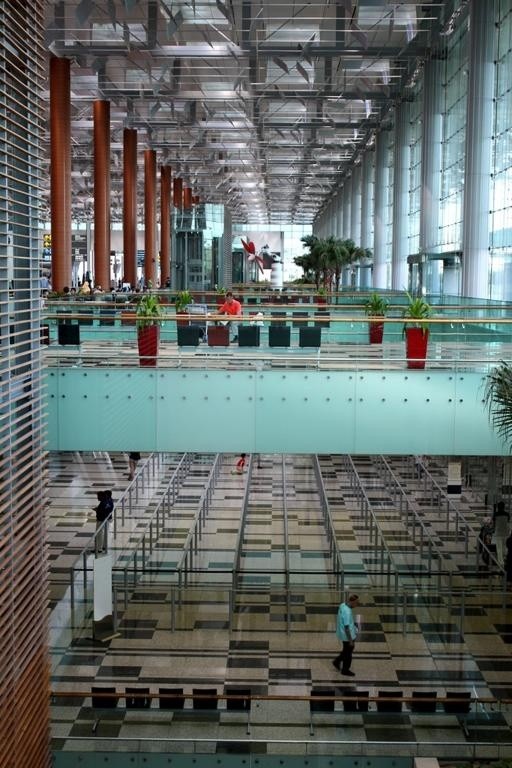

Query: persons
492,500,510,571
91,490,113,552
230,453,246,475
125,452,141,481
39,272,170,298
210,292,244,342
333,595,360,676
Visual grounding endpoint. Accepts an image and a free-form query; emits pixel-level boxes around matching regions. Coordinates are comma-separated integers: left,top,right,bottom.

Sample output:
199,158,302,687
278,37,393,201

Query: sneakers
334,659,340,669
91,547,105,552
231,336,238,342
342,670,355,676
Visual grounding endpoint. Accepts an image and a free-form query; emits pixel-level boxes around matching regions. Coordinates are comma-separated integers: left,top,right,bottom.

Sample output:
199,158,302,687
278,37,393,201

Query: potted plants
317,287,328,310
130,288,193,367
361,287,433,371
215,285,227,310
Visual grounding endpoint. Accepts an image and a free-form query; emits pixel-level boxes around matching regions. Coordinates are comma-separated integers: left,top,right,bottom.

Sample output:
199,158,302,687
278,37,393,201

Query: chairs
39,309,136,365
178,310,331,369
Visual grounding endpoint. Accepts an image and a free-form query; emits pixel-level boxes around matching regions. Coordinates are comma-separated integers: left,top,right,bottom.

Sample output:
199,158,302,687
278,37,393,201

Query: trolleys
187,303,208,343
